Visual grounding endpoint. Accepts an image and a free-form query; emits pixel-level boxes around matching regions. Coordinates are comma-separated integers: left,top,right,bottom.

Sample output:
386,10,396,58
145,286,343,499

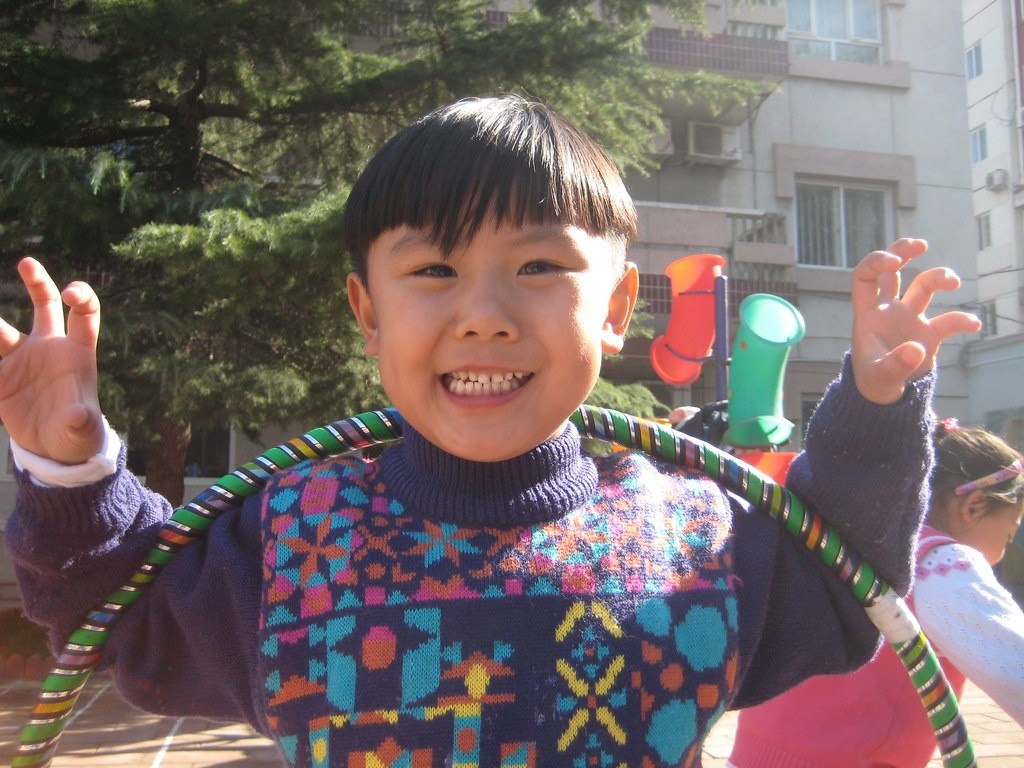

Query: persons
724,417,1024,768
0,91,982,768
